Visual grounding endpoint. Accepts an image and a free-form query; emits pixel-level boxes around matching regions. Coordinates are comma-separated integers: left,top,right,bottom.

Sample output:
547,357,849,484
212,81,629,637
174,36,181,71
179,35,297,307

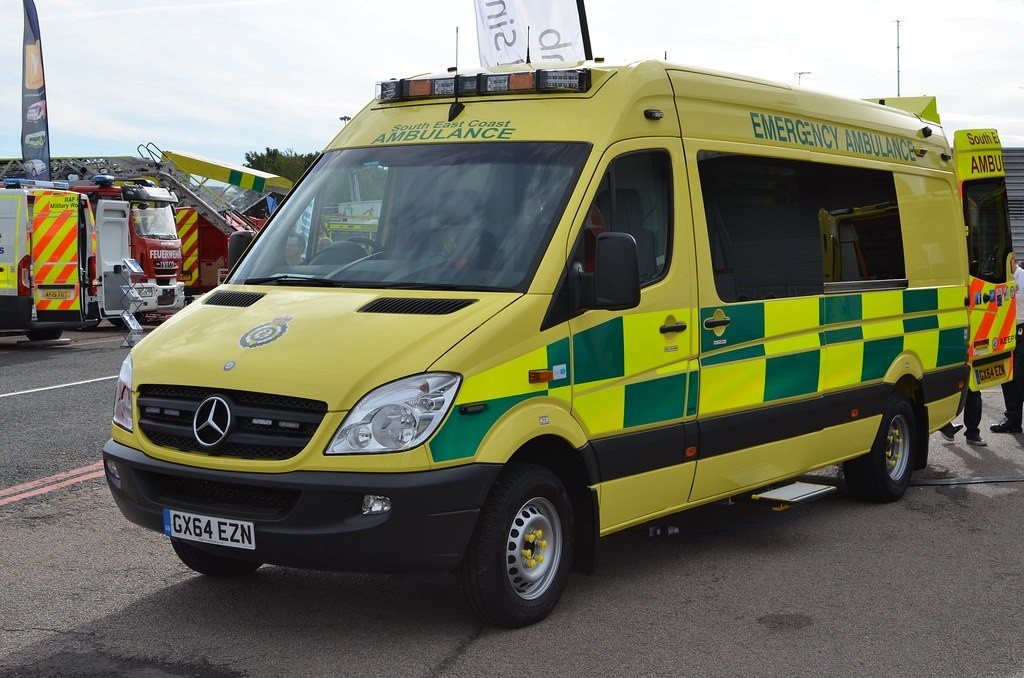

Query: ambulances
101,61,1020,629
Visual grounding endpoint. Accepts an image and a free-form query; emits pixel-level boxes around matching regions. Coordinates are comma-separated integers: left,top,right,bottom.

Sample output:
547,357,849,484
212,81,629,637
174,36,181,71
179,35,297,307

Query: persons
989,254,1024,433
278,233,307,266
938,388,986,446
317,237,334,253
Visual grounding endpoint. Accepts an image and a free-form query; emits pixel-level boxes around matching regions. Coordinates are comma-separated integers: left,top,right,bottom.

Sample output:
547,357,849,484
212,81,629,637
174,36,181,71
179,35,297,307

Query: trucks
0,174,306,340
27,100,45,124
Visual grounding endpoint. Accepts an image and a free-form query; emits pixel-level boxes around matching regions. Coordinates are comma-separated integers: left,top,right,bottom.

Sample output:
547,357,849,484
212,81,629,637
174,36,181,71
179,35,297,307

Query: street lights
339,115,352,126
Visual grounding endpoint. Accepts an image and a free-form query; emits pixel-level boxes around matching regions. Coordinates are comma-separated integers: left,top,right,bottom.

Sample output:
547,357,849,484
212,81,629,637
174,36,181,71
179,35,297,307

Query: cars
23,159,46,176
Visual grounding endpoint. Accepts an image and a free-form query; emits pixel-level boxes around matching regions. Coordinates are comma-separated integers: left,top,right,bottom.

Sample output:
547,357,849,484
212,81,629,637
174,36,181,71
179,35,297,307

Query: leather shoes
990,421,1022,433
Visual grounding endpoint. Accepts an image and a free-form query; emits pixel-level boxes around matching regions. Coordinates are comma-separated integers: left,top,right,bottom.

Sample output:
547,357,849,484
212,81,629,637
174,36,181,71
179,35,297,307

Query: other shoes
966,431,987,446
940,423,954,441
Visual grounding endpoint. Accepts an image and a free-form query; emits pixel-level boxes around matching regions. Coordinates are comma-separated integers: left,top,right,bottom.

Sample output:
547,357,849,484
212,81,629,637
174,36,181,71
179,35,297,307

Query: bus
25,130,45,148
